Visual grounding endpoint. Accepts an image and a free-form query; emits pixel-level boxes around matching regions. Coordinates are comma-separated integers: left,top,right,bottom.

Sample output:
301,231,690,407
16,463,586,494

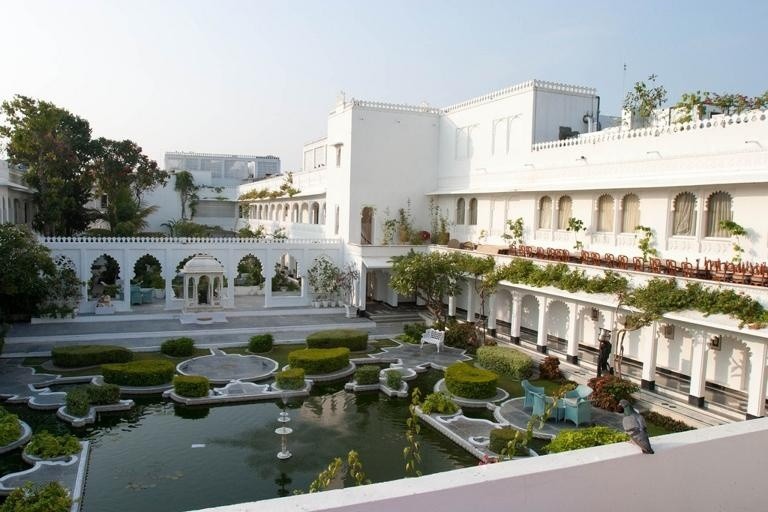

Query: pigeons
617,399,654,455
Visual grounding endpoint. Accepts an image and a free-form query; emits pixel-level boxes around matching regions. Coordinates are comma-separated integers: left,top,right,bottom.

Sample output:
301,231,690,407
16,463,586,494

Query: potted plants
150,257,359,318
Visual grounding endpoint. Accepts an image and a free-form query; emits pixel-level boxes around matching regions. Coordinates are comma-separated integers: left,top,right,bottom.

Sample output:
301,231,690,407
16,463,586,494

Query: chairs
522,379,597,428
119,286,154,306
510,244,768,287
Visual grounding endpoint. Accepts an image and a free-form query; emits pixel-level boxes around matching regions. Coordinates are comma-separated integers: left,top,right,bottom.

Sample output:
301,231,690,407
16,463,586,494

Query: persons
97,289,111,306
596,335,612,377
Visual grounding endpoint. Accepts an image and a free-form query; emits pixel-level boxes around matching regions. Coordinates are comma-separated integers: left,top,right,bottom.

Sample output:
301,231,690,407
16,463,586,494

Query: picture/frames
665,324,674,340
710,336,721,351
591,307,599,321
